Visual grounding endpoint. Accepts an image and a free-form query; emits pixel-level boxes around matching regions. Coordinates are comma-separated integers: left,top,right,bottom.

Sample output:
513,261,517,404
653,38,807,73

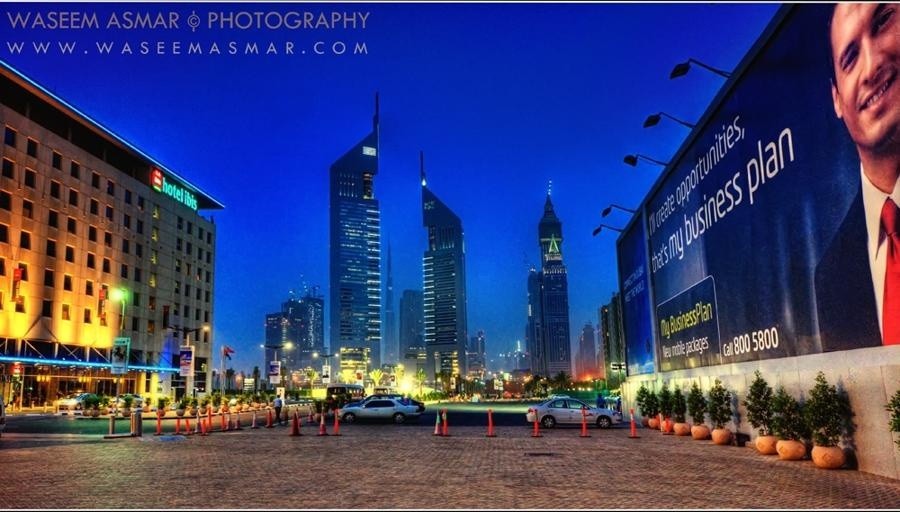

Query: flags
110,337,132,375
97,289,108,318
10,268,23,302
178,345,195,377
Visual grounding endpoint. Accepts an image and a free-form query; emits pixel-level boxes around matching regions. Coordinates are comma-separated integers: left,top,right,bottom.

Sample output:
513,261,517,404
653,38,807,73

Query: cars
526,398,623,428
337,394,424,424
0,396,7,432
54,392,144,410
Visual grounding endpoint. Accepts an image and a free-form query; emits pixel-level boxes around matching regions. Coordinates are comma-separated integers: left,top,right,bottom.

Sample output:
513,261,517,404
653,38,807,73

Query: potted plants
635,370,855,470
81,393,275,418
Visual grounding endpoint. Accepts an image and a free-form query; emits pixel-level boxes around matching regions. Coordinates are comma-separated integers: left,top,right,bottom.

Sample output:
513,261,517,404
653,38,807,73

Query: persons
255,389,299,403
814,4,899,352
616,397,621,411
322,391,352,412
11,389,65,411
595,393,608,408
273,395,282,424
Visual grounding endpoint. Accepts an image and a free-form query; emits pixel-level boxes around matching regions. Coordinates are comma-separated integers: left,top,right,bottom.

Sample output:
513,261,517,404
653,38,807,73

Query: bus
326,383,393,400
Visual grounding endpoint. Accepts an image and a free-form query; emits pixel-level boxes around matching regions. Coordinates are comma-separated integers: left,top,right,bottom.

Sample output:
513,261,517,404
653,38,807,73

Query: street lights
115,289,125,339
519,353,538,373
167,326,209,398
313,352,339,383
591,57,731,237
261,343,291,394
611,361,626,383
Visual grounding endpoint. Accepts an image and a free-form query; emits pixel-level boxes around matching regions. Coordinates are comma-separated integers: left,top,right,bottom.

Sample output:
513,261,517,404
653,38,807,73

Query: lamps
592,224,623,236
602,204,636,217
643,113,695,130
670,59,729,80
623,153,667,167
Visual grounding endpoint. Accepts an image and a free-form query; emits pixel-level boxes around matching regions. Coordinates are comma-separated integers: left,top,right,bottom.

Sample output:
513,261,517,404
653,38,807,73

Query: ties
878,196,900,348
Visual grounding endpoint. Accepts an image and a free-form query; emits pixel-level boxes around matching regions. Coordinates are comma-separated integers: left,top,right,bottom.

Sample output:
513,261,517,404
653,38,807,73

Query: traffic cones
431,406,451,436
154,402,341,436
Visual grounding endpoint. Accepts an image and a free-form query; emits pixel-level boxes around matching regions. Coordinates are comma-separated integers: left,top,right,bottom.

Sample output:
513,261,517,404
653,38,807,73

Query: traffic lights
196,385,205,392
9,266,24,305
356,372,362,380
390,375,395,382
